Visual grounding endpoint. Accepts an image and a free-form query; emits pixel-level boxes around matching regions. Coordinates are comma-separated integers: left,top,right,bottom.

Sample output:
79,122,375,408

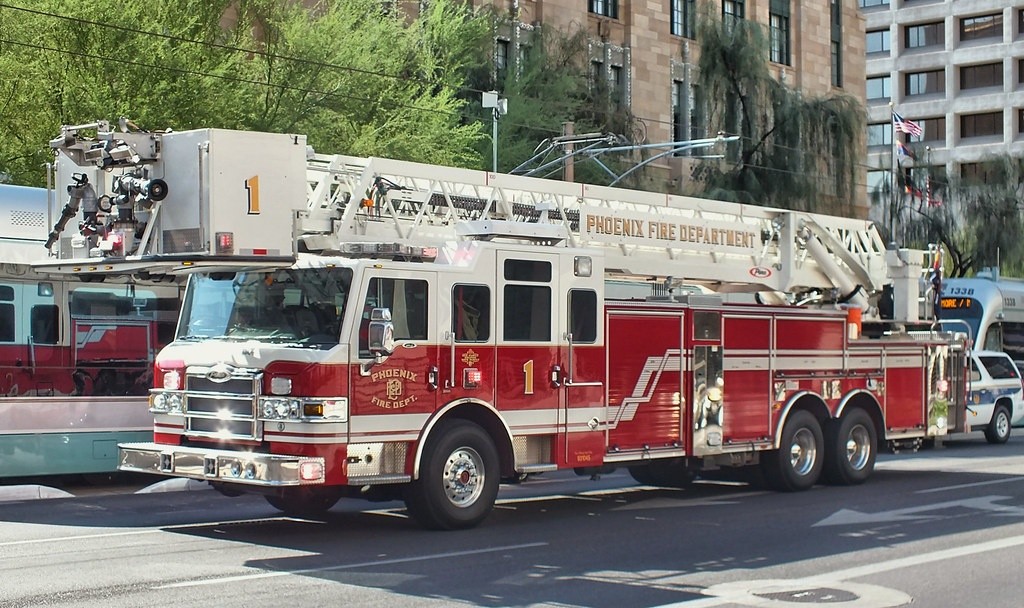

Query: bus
0,183,377,479
940,266,1024,378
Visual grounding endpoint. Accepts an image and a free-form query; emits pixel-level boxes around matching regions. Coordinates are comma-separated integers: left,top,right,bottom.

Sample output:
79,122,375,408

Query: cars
964,339,1024,443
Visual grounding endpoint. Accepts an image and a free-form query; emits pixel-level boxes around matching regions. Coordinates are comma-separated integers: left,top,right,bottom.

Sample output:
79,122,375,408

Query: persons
235,305,255,329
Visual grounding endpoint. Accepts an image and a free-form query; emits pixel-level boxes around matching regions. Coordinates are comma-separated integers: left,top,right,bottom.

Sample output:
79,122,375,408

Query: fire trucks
45,117,973,531
0,282,177,397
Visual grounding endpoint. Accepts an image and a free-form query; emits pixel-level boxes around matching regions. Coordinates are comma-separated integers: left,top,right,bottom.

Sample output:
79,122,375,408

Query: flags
897,157,923,197
926,159,949,207
895,138,918,161
893,110,923,139
